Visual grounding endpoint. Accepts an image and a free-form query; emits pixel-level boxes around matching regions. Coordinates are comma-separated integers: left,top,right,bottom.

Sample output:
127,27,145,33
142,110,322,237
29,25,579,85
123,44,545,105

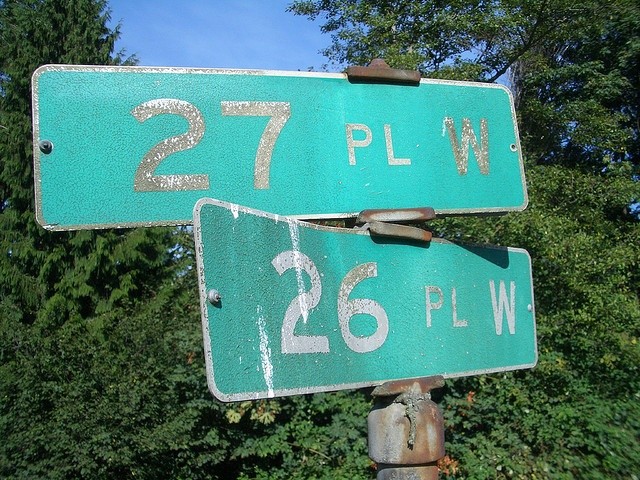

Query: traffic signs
193,198,539,403
30,64,530,233
